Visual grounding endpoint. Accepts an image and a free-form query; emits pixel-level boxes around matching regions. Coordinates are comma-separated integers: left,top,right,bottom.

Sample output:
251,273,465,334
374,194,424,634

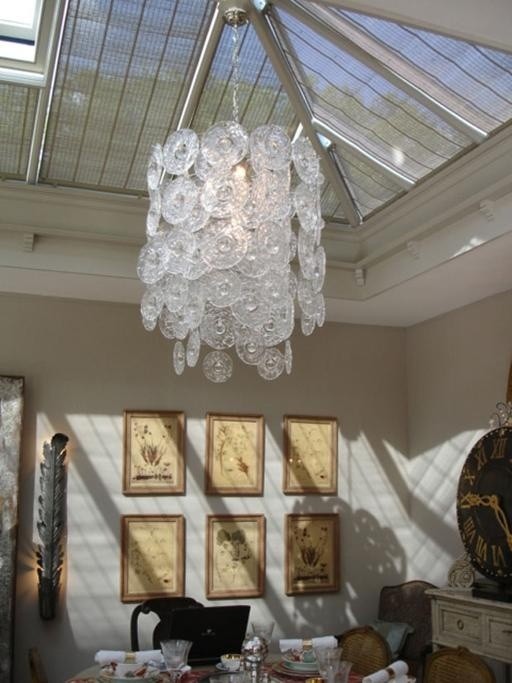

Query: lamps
134,6,331,385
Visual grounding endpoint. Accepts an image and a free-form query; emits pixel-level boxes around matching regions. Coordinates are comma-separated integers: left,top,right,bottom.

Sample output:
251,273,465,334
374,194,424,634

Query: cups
313,645,354,683
250,621,276,645
158,639,193,676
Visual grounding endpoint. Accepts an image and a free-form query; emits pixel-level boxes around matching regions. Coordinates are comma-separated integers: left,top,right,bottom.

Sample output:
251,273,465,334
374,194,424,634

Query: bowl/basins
220,654,244,670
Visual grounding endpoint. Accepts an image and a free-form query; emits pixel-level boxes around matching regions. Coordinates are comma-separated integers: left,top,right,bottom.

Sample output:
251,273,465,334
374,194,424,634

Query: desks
422,586,512,664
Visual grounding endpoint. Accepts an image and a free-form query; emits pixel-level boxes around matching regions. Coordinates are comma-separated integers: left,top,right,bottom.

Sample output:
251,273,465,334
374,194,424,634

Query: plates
99,662,161,681
270,654,332,678
216,662,253,673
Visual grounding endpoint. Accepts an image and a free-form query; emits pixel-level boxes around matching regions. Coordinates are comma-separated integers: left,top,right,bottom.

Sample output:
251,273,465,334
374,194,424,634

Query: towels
94,648,165,667
